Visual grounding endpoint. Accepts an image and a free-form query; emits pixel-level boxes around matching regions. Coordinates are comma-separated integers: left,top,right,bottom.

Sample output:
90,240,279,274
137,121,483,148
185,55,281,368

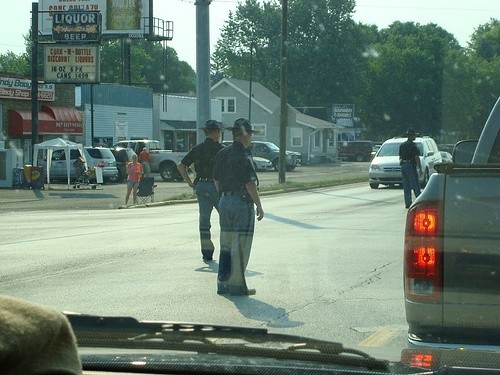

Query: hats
224,117,259,132
405,129,419,135
199,120,224,129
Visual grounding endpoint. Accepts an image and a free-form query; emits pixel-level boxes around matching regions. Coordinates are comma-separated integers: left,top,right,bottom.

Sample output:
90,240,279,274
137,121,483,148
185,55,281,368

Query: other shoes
217,284,256,295
203,249,212,260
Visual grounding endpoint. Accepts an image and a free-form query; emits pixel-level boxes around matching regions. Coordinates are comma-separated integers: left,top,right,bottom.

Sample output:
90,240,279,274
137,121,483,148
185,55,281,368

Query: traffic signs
52,11,102,42
43,42,101,84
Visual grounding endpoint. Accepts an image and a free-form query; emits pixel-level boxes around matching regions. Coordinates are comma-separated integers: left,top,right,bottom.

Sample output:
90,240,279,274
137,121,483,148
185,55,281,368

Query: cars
222,141,302,172
369,136,442,189
253,156,273,172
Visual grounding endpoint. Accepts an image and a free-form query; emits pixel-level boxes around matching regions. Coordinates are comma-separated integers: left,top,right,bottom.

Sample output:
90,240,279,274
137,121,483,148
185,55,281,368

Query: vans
103,148,138,183
38,148,118,184
337,140,373,162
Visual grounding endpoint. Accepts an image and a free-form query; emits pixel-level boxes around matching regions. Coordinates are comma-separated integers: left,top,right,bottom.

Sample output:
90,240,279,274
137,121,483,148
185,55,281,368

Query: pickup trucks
401,98,500,372
110,140,189,182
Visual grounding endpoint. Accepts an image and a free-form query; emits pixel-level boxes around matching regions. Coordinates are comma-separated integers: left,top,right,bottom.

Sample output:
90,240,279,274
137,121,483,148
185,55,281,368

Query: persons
399,128,423,209
179,120,228,262
216,117,264,296
139,147,151,177
125,155,142,205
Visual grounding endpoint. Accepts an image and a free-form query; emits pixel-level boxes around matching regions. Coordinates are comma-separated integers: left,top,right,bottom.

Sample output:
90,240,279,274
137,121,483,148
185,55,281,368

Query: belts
400,159,413,163
197,177,213,182
222,191,247,196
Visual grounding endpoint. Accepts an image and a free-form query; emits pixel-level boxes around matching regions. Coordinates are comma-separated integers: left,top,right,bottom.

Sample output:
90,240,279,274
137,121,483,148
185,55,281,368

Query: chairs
136,177,157,204
